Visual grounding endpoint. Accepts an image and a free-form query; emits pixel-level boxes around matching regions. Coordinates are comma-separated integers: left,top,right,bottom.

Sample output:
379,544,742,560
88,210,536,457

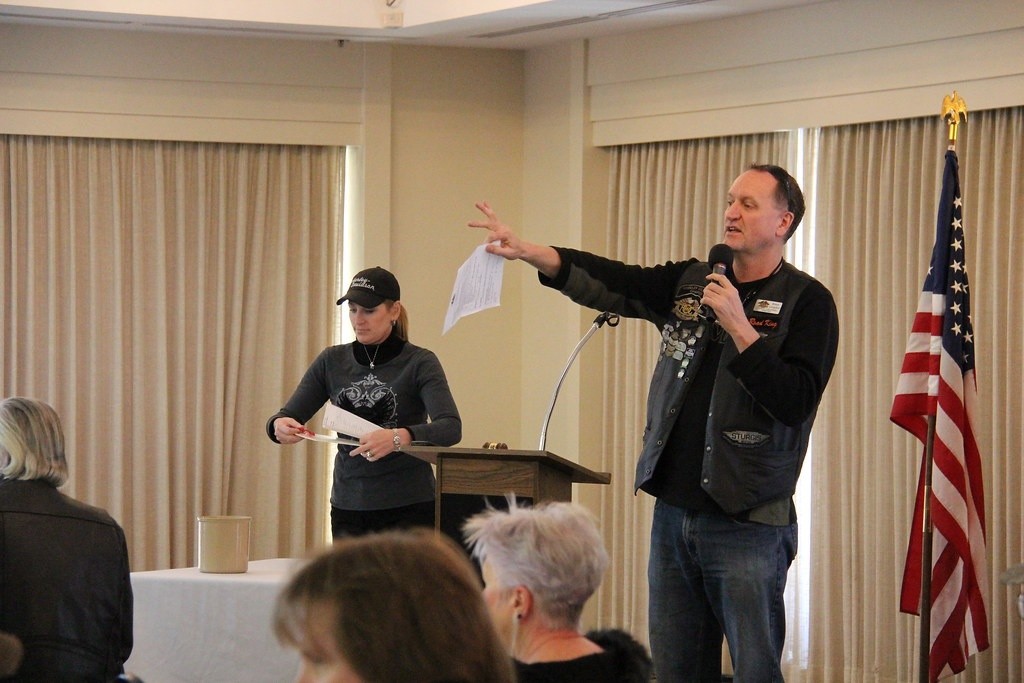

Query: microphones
706,244,734,323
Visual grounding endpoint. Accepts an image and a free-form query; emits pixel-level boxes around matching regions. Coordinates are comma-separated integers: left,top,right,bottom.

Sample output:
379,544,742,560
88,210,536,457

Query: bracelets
392,427,402,452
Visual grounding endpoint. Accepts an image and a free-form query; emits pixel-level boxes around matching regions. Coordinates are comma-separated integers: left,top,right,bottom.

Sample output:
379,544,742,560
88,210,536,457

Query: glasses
695,292,749,323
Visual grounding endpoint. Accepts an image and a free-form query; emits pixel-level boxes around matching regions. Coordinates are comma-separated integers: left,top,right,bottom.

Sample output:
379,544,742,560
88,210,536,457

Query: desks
123,557,304,683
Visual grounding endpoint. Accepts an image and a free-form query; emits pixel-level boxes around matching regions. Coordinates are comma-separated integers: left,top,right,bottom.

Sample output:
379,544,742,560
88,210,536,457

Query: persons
471,502,656,683
269,530,519,683
0,397,136,683
265,265,464,547
465,163,838,683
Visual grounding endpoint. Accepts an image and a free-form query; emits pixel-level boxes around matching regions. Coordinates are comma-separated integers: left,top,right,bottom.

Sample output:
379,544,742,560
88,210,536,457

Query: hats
336,266,401,309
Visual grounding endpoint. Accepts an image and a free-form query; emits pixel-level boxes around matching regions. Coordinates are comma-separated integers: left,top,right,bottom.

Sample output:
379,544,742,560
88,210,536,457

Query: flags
889,152,998,678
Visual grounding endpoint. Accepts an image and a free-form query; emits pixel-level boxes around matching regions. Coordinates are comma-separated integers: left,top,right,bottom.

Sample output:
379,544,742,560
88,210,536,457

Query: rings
366,450,372,459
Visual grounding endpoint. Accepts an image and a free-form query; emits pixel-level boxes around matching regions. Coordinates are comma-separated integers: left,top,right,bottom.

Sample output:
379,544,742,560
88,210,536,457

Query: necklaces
361,343,380,368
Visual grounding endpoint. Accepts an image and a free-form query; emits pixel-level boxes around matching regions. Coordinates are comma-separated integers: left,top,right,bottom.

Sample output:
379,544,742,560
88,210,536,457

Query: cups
196,516,252,573
